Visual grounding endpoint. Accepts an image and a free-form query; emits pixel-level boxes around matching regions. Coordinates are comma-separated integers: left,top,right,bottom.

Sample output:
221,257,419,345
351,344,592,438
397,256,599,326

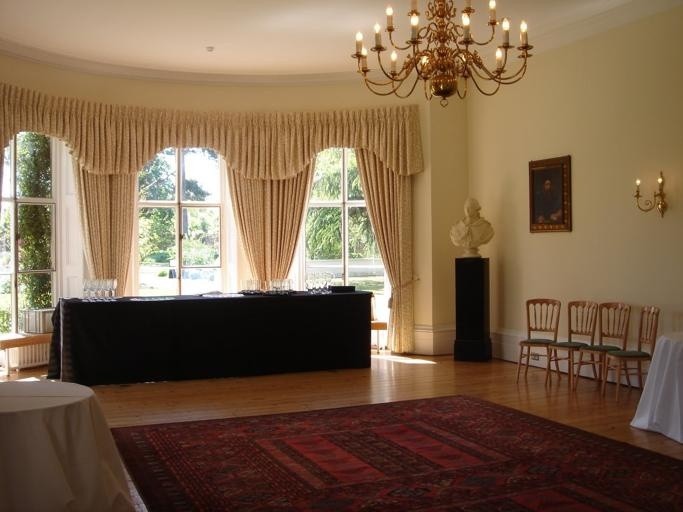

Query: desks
60,290,374,387
0,380,96,512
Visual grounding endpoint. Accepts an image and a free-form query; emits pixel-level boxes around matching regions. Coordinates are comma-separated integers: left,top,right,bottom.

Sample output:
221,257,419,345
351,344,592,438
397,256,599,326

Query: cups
242,280,291,295
306,277,334,295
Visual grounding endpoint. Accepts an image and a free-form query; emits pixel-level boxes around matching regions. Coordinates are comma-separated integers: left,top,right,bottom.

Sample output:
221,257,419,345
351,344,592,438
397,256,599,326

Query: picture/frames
529,155,572,233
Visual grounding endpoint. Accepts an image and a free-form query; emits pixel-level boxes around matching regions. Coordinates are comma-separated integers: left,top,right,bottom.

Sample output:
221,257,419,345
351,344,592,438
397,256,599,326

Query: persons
533,176,563,225
447,196,493,249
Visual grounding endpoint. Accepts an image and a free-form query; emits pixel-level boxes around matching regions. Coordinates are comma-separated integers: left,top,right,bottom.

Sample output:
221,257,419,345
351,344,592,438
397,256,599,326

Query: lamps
351,0,533,109
634,170,668,218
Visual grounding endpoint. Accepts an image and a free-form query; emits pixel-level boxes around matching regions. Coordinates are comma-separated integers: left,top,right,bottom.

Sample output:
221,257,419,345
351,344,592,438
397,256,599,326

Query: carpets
110,395,682,511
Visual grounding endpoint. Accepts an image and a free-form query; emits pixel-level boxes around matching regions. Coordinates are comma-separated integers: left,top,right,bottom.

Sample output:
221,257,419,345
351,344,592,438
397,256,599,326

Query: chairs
516,298,660,402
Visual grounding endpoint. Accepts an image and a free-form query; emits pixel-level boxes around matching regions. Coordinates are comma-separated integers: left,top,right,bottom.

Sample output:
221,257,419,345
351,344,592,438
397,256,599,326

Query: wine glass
78,277,119,303
